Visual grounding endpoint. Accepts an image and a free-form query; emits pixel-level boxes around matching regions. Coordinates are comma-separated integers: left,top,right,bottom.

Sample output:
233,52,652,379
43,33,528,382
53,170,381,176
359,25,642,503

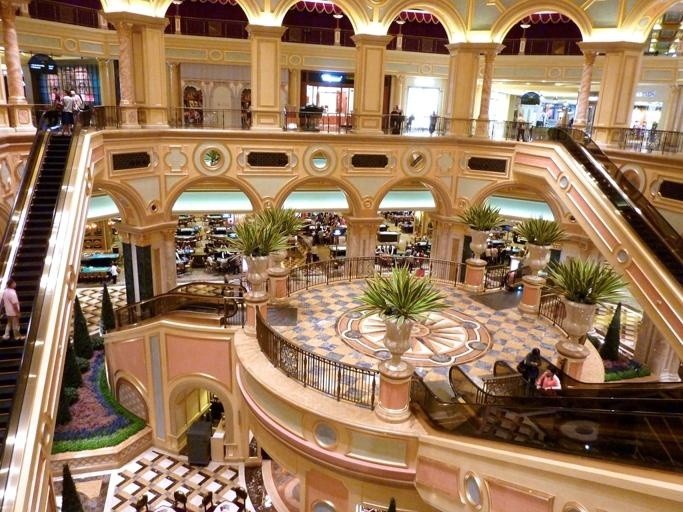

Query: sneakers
3,335,25,340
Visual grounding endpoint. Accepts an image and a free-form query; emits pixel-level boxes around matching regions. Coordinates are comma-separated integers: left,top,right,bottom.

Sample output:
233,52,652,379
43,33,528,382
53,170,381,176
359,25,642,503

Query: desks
78,252,119,281
176,214,234,274
378,214,430,250
308,225,347,252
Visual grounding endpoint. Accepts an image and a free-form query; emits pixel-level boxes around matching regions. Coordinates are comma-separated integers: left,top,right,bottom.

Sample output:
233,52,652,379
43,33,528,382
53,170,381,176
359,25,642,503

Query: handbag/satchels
517,361,528,373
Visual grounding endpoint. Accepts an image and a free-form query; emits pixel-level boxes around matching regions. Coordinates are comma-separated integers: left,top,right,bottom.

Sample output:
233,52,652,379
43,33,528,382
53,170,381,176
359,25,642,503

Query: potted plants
549,253,630,352
352,265,450,372
462,205,564,284
226,204,303,300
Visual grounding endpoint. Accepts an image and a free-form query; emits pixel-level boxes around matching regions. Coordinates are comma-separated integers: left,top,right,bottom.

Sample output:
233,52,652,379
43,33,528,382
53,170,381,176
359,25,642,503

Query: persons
390,106,404,136
301,211,347,271
109,262,119,282
246,101,252,128
375,211,432,273
516,114,528,141
524,348,542,394
430,112,441,132
2,279,25,339
537,365,561,390
485,232,514,265
51,84,82,133
177,215,242,275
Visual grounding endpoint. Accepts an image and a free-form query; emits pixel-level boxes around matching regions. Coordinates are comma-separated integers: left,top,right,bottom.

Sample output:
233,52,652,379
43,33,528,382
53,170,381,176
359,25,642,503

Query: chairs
135,486,247,511
